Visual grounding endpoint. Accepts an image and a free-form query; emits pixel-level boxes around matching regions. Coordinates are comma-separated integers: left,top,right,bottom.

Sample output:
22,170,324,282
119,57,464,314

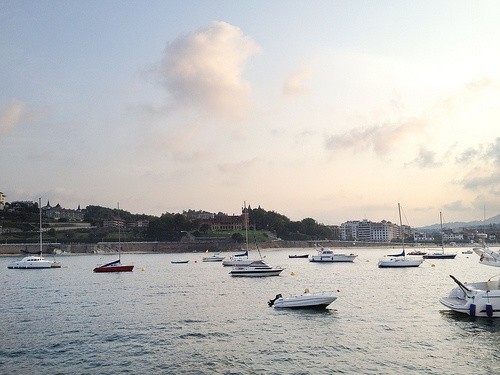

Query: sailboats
423,211,457,258
92,201,137,273
8,196,63,269
221,202,266,266
377,202,424,267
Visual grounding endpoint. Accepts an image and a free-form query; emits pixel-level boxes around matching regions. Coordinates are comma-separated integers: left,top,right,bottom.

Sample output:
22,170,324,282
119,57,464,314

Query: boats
437,274,499,317
307,249,358,262
473,246,500,266
463,250,472,254
407,249,426,255
269,288,339,310
202,254,225,262
289,254,309,258
228,260,283,277
171,259,190,263
